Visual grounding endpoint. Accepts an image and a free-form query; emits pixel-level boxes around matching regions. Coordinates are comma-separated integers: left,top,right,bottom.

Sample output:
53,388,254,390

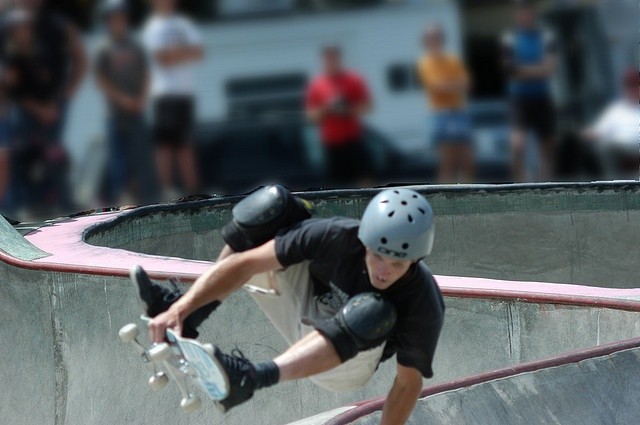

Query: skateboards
119,312,231,408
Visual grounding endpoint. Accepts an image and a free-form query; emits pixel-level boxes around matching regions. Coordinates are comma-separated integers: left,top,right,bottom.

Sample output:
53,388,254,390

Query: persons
0,1,86,216
141,0,209,204
128,185,447,424
303,43,379,189
416,22,479,186
552,68,640,183
90,0,150,207
497,0,562,184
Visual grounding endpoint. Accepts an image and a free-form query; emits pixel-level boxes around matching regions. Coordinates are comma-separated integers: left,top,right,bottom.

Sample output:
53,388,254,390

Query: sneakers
204,343,256,411
132,267,199,339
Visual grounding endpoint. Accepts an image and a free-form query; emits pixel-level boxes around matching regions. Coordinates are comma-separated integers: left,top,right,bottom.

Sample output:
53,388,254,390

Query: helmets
358,188,436,263
